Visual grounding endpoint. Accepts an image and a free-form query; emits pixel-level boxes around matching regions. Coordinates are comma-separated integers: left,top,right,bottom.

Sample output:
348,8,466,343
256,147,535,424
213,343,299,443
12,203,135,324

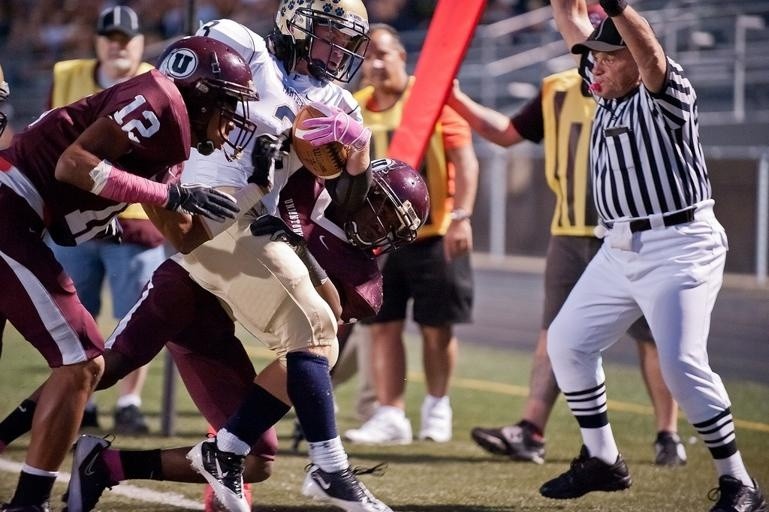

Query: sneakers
79,406,103,435
652,429,689,466
113,404,150,436
60,433,118,512
471,422,548,467
343,404,414,445
183,432,251,512
300,461,394,512
417,401,454,443
539,444,633,501
706,473,769,512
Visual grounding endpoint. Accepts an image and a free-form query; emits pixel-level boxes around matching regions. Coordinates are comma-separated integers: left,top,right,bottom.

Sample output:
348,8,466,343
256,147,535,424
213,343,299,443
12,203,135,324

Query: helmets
269,0,372,85
154,35,260,163
343,156,431,261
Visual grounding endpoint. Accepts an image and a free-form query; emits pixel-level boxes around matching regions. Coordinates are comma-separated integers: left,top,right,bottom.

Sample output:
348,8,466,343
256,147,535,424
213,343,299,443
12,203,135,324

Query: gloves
246,132,290,195
163,180,241,223
248,212,309,251
301,99,374,153
96,216,124,247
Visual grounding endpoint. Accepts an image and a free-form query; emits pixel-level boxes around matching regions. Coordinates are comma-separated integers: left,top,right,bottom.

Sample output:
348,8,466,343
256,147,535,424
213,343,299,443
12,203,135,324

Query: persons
0,64,12,141
0,36,262,512
443,4,689,467
538,0,765,511
333,70,379,423
176,1,397,512
341,22,481,448
48,6,164,435
1,156,429,510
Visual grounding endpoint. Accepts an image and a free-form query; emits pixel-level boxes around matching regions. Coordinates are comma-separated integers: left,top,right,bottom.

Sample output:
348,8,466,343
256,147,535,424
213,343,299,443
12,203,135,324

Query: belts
601,208,699,234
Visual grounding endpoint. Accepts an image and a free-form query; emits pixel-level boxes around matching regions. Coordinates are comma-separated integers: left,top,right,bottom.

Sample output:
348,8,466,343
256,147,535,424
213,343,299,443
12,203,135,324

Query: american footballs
292,105,347,180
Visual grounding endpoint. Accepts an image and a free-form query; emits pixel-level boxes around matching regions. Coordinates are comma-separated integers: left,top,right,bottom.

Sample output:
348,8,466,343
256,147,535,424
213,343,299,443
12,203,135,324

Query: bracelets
597,0,628,18
450,210,470,221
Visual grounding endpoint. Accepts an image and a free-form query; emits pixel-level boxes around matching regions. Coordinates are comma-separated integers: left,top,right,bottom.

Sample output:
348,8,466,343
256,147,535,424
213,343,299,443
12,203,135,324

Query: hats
93,5,144,38
571,13,656,54
